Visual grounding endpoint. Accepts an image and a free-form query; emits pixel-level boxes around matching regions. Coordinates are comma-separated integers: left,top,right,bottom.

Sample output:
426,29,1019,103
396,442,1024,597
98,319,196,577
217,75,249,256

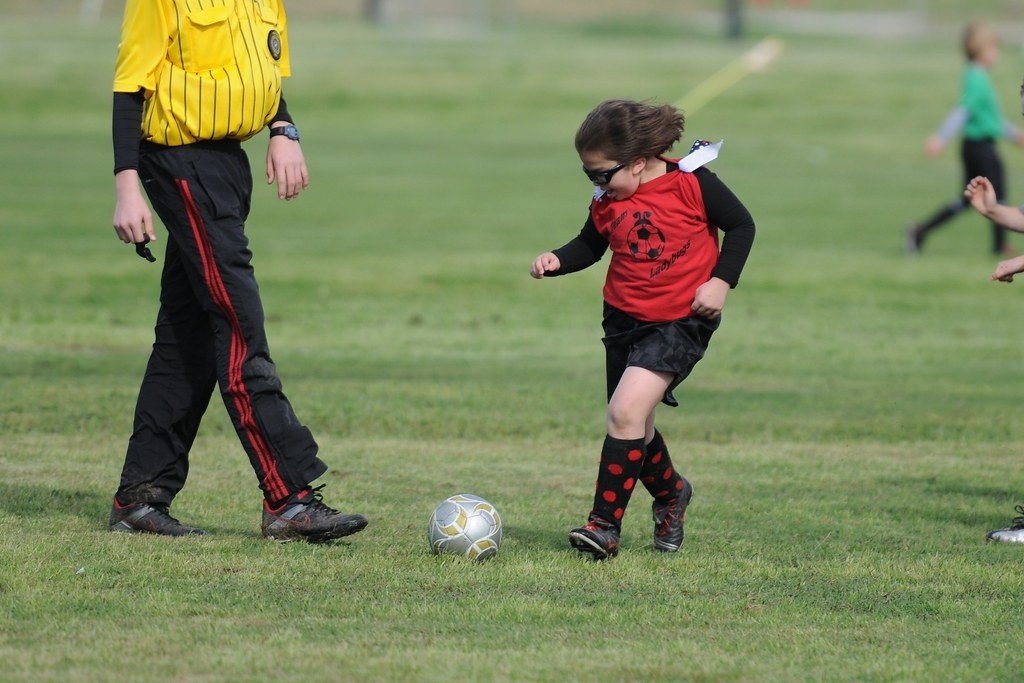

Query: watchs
270,125,300,140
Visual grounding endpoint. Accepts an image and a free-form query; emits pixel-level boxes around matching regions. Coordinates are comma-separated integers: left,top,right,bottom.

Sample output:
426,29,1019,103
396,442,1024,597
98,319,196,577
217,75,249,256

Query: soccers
427,492,503,562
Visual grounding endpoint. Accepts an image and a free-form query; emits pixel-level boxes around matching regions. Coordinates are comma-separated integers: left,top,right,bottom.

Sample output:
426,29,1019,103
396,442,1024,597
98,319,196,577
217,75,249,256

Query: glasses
582,160,631,187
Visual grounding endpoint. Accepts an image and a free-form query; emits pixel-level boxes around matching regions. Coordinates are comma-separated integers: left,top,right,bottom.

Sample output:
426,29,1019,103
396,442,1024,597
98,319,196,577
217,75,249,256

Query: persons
908,20,1024,282
529,100,756,557
108,0,368,547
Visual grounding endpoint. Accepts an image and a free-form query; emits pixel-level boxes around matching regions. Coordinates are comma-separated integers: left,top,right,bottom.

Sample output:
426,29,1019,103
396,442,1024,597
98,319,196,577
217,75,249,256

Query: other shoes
998,246,1018,257
905,228,920,252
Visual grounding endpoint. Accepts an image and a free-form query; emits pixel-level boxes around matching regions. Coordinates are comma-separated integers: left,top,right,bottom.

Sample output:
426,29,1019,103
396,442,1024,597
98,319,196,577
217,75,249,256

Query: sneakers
653,475,694,554
109,496,208,537
568,521,619,561
262,482,368,543
